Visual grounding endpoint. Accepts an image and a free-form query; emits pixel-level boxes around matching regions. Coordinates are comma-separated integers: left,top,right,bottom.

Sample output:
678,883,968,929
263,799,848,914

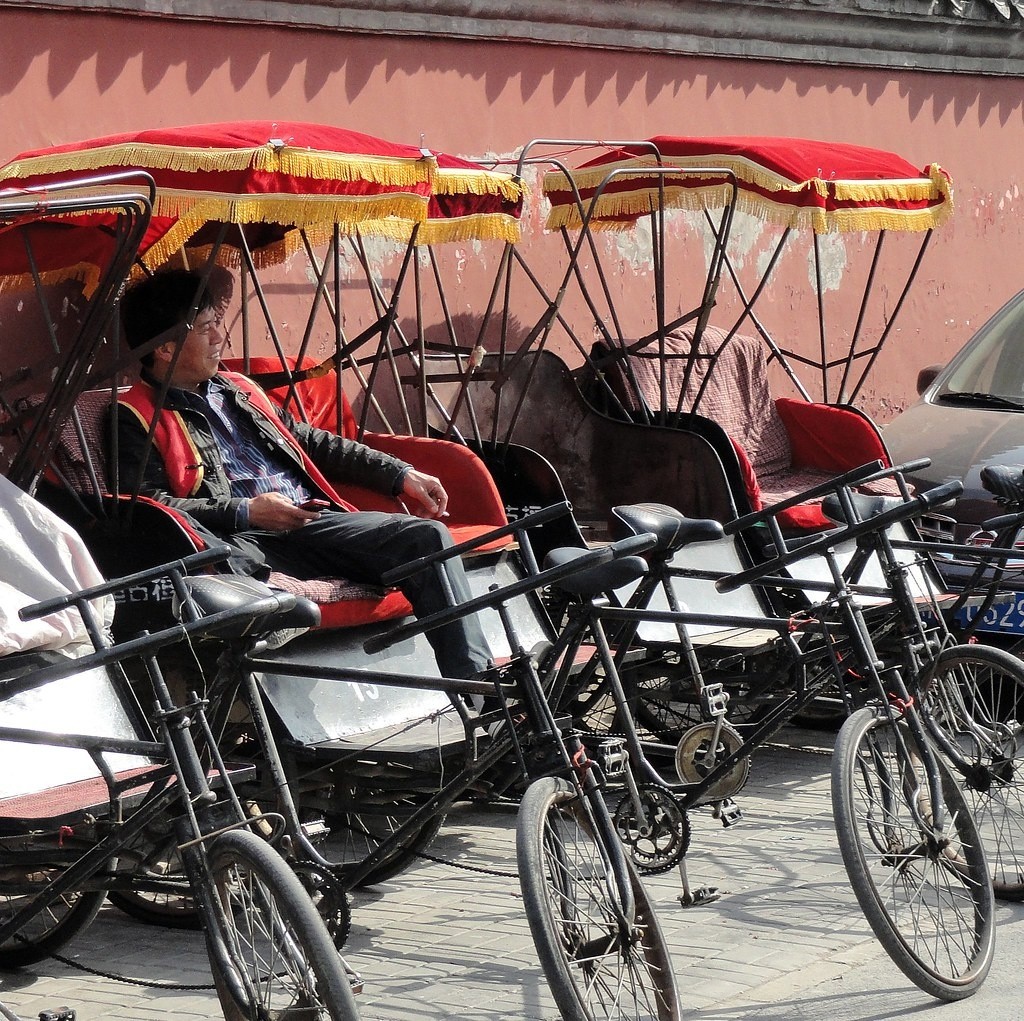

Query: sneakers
251,628,310,649
460,640,554,743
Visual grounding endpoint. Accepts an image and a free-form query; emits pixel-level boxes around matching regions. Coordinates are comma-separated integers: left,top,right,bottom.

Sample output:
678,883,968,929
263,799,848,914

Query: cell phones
297,499,331,512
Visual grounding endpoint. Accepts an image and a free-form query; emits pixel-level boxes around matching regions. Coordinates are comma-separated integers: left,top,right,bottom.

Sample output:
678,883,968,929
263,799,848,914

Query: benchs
580,326,899,533
214,357,515,550
19,382,415,628
406,350,742,552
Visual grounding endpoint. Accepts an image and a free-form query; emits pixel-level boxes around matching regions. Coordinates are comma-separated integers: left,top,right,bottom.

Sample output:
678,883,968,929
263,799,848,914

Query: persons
97,263,552,741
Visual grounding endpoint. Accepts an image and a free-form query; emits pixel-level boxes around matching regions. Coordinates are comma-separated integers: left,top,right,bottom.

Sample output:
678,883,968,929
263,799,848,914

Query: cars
882,288,1024,716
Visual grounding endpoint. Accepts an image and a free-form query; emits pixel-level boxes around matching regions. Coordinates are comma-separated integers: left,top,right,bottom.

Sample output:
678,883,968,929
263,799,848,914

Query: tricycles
1,115,1024,1021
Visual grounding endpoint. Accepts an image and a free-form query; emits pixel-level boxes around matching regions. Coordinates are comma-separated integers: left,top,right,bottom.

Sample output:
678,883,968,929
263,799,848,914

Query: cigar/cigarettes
438,510,451,518
